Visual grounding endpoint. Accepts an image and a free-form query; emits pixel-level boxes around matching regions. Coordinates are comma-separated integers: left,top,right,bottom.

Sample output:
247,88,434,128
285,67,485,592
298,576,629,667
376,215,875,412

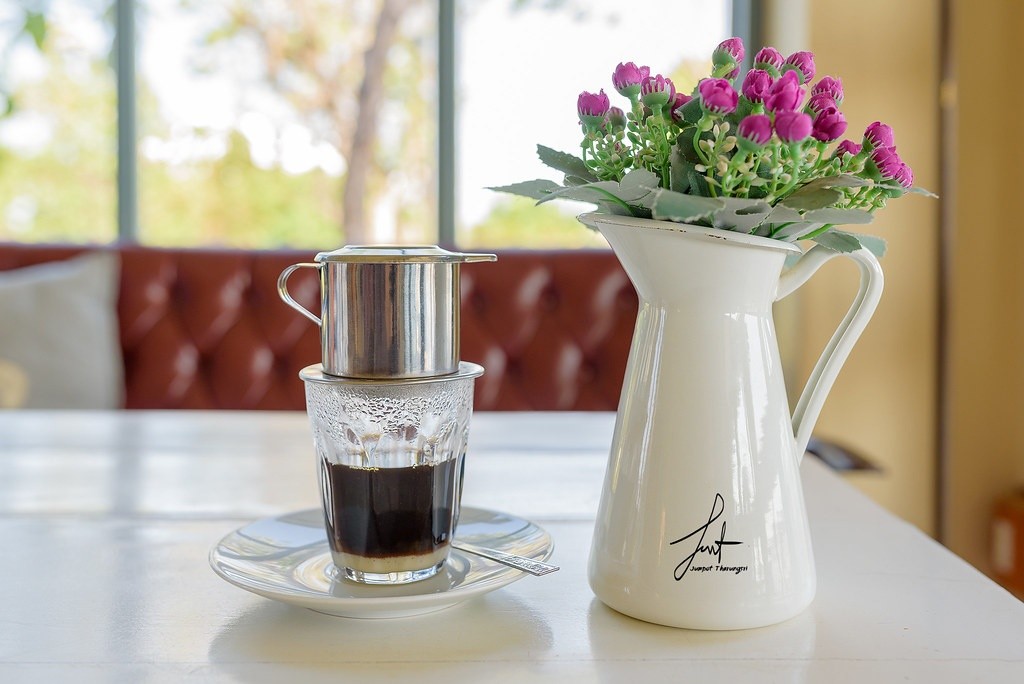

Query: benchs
3,240,637,412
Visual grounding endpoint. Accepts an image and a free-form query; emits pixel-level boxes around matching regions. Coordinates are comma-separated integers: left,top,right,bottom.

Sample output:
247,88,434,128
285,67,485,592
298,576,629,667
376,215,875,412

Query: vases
579,214,885,631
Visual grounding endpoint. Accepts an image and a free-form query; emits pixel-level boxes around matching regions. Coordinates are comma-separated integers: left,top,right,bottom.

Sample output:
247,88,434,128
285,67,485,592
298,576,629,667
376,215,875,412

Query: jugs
277,246,496,381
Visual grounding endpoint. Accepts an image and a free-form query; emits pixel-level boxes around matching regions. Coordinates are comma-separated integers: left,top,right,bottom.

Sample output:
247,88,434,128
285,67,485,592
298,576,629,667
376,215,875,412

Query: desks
0,410,1023,684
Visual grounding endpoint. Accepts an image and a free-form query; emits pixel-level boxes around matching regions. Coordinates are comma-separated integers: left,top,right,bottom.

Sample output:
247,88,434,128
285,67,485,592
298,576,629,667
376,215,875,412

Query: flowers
486,39,939,255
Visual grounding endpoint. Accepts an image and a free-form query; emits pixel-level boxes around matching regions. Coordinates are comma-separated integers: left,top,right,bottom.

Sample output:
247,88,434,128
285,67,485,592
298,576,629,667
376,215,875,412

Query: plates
210,507,554,618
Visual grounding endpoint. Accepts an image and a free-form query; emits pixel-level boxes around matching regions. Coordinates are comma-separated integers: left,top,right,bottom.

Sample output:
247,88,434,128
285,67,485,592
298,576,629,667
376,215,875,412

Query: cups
301,376,473,583
